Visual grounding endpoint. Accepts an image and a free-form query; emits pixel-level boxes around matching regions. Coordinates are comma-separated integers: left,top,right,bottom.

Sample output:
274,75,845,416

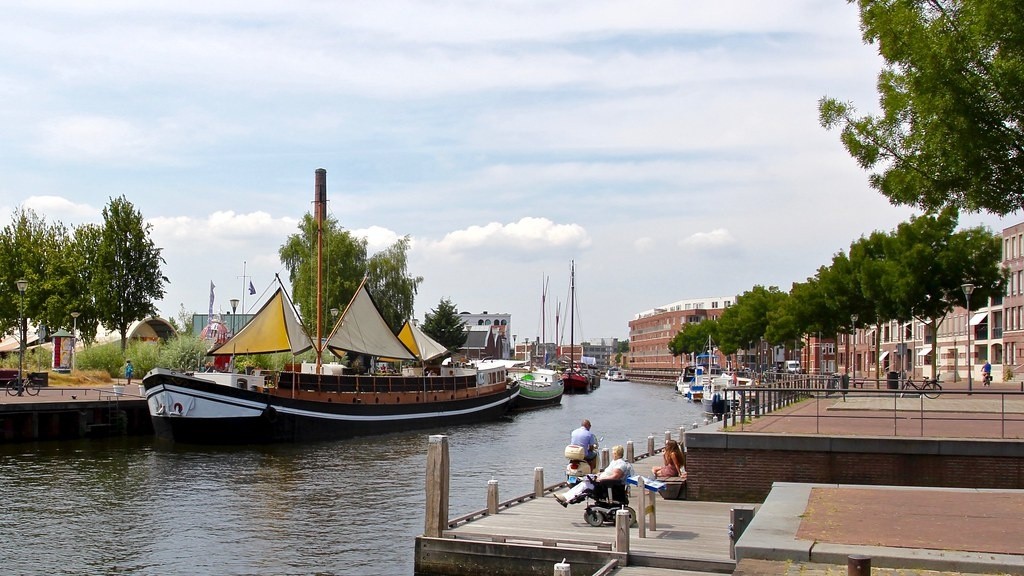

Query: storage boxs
564,444,585,461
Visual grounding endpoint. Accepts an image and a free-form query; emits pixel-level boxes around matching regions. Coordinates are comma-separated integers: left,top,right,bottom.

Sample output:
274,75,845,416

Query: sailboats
555,258,601,395
505,270,566,413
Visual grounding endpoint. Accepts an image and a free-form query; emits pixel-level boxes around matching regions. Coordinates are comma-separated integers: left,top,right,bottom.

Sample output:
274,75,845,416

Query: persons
645,440,686,496
981,362,990,381
740,366,783,384
380,364,388,372
447,361,475,369
570,420,597,473
448,370,455,377
553,445,629,508
124,359,134,385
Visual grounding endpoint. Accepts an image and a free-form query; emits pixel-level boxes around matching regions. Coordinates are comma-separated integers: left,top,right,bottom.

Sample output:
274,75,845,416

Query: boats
138,166,524,449
674,334,758,421
604,365,626,381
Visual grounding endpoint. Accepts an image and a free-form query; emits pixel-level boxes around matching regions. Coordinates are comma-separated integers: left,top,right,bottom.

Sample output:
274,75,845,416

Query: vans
783,360,801,375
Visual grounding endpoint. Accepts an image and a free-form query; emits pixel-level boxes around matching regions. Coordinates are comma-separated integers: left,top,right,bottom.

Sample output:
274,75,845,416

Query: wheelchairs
555,472,636,528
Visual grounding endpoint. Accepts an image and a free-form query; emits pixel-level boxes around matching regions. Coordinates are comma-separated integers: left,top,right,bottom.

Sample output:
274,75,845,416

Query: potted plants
111,357,125,397
137,362,146,396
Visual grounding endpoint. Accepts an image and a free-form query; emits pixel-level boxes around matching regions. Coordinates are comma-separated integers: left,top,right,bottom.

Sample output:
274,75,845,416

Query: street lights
960,283,975,396
849,312,859,388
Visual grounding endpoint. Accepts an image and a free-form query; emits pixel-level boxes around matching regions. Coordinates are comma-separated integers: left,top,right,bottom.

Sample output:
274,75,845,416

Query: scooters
562,436,604,489
981,370,992,387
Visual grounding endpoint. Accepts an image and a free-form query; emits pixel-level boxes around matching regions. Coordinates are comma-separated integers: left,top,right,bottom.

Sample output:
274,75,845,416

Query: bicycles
899,376,942,400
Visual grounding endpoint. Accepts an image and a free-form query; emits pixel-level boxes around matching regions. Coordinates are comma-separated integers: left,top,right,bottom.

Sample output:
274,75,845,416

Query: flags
249,281,257,295
207,281,216,338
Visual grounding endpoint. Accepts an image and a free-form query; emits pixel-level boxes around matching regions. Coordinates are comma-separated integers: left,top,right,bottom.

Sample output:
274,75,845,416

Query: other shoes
553,492,567,502
556,498,568,508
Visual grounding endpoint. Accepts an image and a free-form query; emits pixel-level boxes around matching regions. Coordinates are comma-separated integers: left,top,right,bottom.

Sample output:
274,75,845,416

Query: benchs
854,376,867,387
0,376,44,386
655,476,688,500
